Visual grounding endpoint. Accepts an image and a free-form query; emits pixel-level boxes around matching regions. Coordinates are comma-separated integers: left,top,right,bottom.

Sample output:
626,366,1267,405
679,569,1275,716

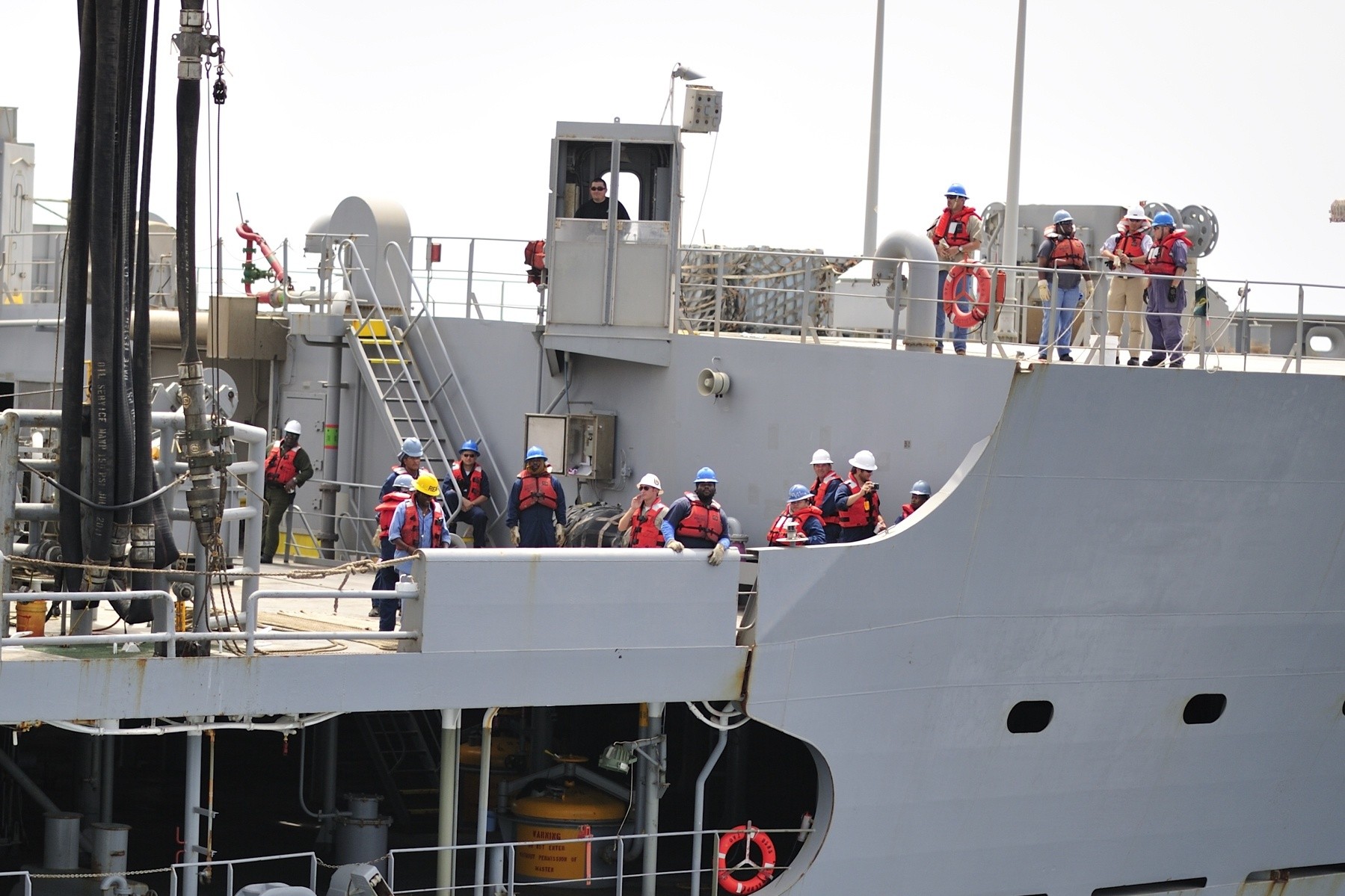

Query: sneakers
935,347,1184,368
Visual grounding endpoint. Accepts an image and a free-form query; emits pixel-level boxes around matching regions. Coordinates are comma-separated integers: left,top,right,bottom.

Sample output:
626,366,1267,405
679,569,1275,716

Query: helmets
909,479,932,496
1053,209,1074,225
1123,203,1174,227
459,439,481,457
524,446,548,463
847,450,878,471
636,473,664,495
786,484,816,503
694,467,720,484
810,449,834,465
401,436,424,457
393,474,440,496
284,420,302,435
944,184,970,200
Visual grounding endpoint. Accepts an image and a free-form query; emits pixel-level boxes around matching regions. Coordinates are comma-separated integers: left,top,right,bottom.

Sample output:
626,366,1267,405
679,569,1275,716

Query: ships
0,0,1345,896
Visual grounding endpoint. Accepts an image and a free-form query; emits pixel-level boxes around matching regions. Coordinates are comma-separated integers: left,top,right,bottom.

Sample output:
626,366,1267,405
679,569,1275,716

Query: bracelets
1129,257,1132,264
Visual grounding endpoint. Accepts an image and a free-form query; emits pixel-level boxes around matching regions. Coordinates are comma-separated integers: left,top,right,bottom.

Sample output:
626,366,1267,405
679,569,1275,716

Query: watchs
958,245,964,252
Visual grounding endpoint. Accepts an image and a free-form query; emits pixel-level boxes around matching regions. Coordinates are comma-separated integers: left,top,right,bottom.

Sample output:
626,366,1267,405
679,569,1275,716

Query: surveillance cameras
672,63,705,81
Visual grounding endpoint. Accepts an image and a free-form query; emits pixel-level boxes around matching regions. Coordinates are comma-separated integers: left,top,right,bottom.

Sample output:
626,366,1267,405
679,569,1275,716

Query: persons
894,480,932,524
573,179,631,242
1098,204,1156,365
441,440,492,549
376,472,423,632
768,483,826,547
805,448,848,543
258,419,315,564
1142,211,1190,367
661,467,732,567
928,184,985,356
617,472,675,549
834,449,887,547
505,445,569,547
368,436,433,618
389,473,450,614
1034,209,1095,360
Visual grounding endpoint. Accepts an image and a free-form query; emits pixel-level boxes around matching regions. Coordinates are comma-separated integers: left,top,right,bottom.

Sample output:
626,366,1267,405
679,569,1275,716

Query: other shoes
368,607,381,617
260,553,273,563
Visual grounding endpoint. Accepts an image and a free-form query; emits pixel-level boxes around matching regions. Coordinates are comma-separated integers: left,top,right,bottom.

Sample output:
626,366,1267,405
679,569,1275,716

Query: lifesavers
716,823,777,895
941,258,993,331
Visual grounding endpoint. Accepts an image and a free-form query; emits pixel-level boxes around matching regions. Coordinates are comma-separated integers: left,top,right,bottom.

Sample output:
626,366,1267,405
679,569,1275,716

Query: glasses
590,187,606,191
639,486,654,491
1130,219,1141,222
464,454,475,458
